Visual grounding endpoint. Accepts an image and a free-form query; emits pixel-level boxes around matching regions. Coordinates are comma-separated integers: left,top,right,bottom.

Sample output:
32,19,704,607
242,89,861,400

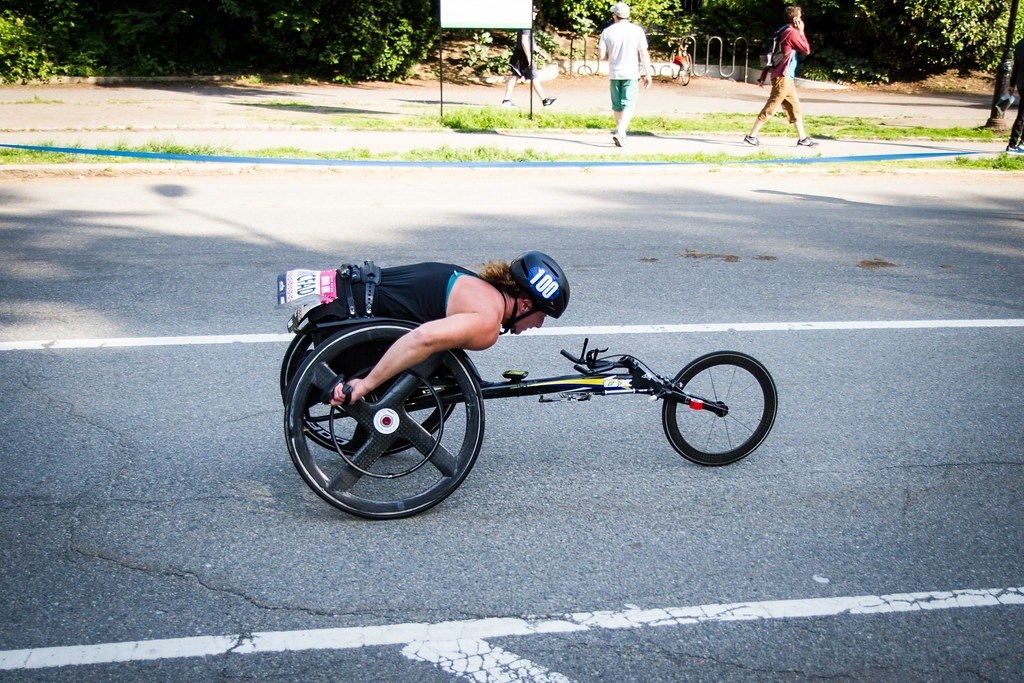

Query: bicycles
670,36,692,85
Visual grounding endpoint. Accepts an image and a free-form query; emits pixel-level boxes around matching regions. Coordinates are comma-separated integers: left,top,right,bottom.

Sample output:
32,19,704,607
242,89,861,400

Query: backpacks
759,25,790,68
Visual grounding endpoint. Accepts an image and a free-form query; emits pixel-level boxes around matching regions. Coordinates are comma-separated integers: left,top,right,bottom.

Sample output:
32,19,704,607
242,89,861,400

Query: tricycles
279,317,779,520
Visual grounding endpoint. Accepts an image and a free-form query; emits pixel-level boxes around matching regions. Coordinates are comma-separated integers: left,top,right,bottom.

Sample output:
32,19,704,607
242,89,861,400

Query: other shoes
797,136,820,147
542,97,557,108
1006,145,1016,153
613,134,624,147
1015,145,1024,153
743,134,763,147
501,100,516,106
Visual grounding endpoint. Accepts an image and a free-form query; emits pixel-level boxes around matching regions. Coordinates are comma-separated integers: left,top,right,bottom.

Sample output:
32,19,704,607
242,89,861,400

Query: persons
744,7,818,147
308,251,570,404
502,6,556,106
598,3,651,147
1005,38,1024,155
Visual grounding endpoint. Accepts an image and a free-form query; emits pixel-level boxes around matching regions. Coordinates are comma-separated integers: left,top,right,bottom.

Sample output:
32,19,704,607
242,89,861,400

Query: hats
532,5,540,13
610,2,630,18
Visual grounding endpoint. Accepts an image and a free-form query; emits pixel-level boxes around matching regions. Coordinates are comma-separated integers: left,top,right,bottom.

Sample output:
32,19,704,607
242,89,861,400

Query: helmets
511,251,572,319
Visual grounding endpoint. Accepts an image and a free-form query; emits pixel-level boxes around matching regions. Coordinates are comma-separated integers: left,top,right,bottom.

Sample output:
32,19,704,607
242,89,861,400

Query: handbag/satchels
995,93,1015,116
